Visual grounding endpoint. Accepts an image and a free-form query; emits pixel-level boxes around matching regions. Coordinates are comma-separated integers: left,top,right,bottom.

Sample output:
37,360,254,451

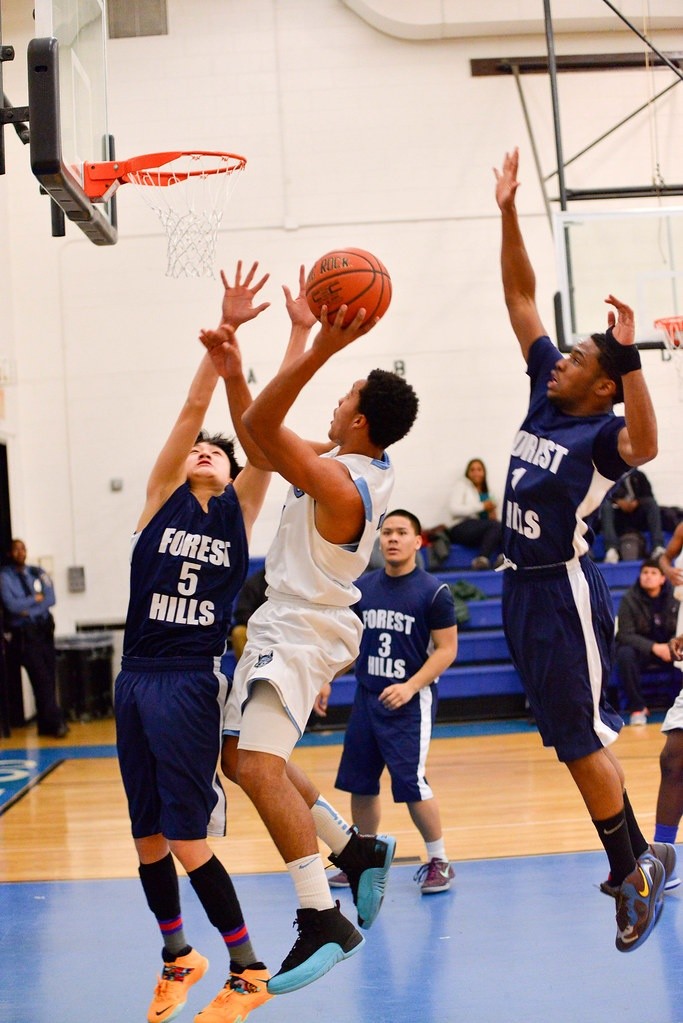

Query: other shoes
664,872,681,889
630,712,646,725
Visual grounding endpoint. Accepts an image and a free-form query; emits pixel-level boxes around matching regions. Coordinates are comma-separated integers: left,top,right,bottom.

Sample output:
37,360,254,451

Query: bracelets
613,344,642,376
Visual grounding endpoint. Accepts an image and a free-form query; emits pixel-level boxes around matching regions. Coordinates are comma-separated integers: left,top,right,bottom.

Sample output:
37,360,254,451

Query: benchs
221,528,678,708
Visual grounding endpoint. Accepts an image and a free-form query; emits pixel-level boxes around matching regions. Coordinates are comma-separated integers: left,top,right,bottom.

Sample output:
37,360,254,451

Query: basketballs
305,247,392,328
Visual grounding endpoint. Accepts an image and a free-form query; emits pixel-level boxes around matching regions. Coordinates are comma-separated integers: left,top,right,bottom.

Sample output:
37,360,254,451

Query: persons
0,539,69,737
447,459,504,569
116,259,317,1023
200,303,418,996
313,509,457,893
617,521,683,726
655,688,683,889
594,470,665,564
492,148,677,953
232,572,267,660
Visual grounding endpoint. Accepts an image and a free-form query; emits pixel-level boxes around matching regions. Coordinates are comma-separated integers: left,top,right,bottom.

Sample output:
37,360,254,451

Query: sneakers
600,843,676,926
328,823,397,930
266,900,366,995
194,960,275,1023
328,871,350,887
616,855,665,952
414,857,455,893
148,945,209,1023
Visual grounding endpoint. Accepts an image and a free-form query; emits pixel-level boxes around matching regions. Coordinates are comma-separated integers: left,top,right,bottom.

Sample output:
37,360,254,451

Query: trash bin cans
55,633,114,722
